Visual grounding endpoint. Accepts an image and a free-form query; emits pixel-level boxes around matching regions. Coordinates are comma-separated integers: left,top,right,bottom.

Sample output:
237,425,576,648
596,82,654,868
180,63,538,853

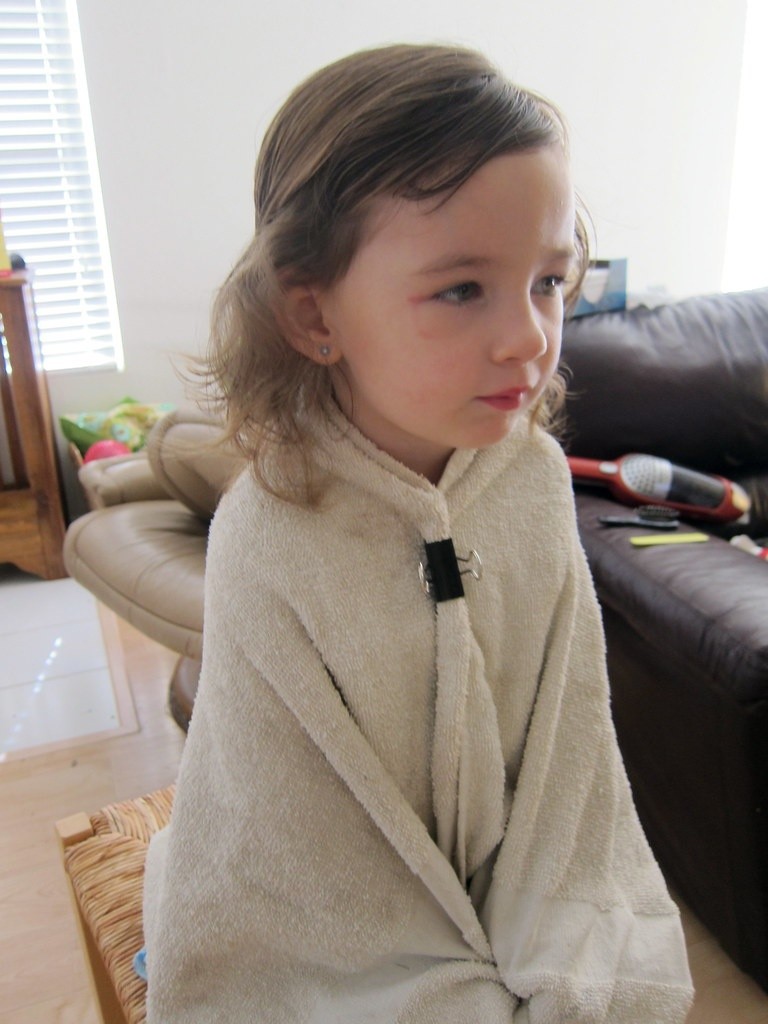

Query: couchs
543,287,767,1004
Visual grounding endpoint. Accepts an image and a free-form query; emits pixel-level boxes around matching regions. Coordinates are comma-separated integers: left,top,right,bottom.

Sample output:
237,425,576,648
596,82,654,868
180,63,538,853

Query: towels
141,378,694,1023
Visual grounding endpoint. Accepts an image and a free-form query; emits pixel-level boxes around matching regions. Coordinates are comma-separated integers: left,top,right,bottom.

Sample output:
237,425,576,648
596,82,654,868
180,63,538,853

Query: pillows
58,394,159,456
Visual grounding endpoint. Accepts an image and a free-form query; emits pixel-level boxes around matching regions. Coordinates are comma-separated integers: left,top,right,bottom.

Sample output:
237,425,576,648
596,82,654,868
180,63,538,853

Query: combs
592,505,681,533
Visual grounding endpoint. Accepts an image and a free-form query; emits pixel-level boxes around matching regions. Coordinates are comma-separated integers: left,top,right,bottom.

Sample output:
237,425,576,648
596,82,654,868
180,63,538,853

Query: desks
0,265,70,580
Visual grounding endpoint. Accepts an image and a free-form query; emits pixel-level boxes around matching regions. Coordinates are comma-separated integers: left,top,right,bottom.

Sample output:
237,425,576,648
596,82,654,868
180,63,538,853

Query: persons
150,45,700,1024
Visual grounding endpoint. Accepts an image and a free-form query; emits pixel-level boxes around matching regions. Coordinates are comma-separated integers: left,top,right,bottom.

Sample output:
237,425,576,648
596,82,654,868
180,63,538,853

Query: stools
55,780,178,1024
